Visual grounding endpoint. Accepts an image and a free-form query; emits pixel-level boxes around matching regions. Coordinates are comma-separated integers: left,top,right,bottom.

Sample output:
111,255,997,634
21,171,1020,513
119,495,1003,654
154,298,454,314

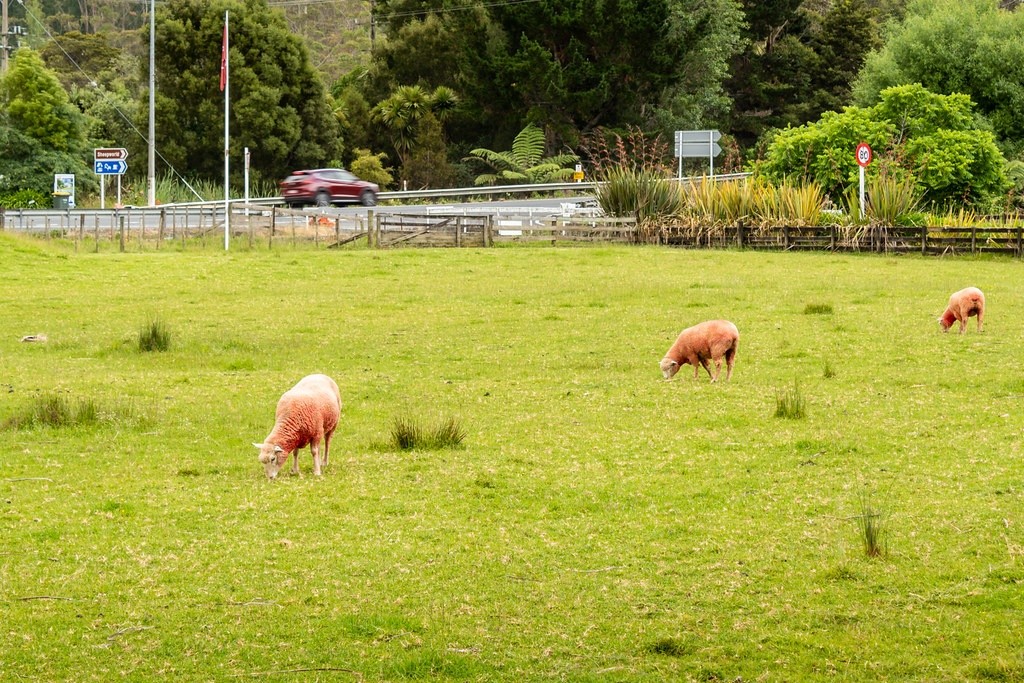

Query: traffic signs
94,160,128,175
94,148,128,160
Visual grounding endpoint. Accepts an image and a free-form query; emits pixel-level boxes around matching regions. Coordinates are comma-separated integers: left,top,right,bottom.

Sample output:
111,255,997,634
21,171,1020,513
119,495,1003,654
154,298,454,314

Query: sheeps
939,286,985,335
657,320,739,383
252,374,343,480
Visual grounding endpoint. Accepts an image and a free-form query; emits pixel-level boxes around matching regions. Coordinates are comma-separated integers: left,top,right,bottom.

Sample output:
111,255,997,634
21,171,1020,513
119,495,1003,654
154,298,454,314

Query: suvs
278,169,379,209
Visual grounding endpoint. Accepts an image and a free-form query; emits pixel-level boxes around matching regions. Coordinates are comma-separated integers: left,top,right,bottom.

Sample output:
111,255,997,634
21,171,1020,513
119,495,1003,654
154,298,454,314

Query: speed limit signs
855,142,872,166
